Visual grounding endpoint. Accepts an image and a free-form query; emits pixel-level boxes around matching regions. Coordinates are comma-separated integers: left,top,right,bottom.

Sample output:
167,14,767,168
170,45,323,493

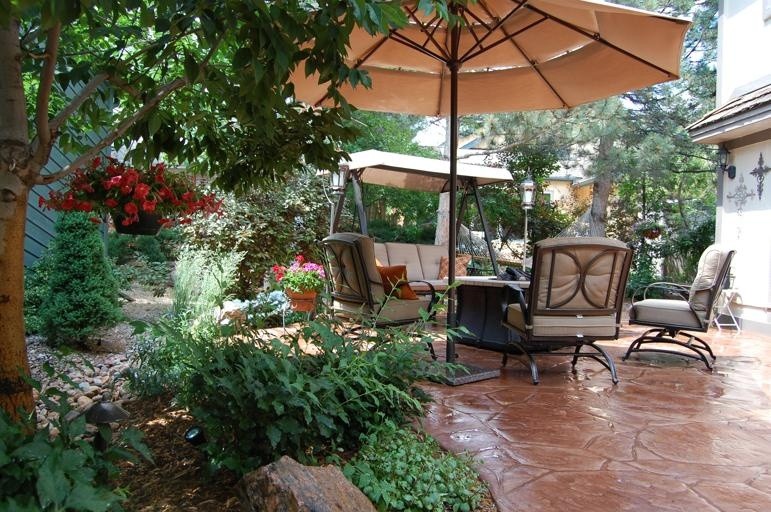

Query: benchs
374,242,468,313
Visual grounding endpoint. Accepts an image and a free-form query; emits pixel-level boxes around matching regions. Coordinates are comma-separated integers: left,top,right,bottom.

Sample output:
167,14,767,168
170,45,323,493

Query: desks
444,275,529,367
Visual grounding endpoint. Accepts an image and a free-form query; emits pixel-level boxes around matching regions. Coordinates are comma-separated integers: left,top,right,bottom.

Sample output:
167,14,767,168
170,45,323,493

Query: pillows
438,256,471,280
376,264,418,300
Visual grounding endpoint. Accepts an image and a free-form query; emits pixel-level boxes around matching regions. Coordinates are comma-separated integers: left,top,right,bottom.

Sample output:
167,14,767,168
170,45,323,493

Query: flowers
37,149,227,230
270,255,325,296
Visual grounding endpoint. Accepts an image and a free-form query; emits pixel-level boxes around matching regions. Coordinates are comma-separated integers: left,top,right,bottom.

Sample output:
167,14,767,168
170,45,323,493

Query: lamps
716,142,737,179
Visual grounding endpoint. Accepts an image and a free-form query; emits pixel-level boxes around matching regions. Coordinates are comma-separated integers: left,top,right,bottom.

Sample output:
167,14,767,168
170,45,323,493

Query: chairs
625,245,735,372
500,237,632,386
323,233,437,360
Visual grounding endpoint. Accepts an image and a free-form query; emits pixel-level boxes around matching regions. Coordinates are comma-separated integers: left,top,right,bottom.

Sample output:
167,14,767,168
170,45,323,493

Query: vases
287,287,316,318
108,205,165,237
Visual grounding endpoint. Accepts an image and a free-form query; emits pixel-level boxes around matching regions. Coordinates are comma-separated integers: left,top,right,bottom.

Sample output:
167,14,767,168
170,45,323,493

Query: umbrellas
248,0,693,363
